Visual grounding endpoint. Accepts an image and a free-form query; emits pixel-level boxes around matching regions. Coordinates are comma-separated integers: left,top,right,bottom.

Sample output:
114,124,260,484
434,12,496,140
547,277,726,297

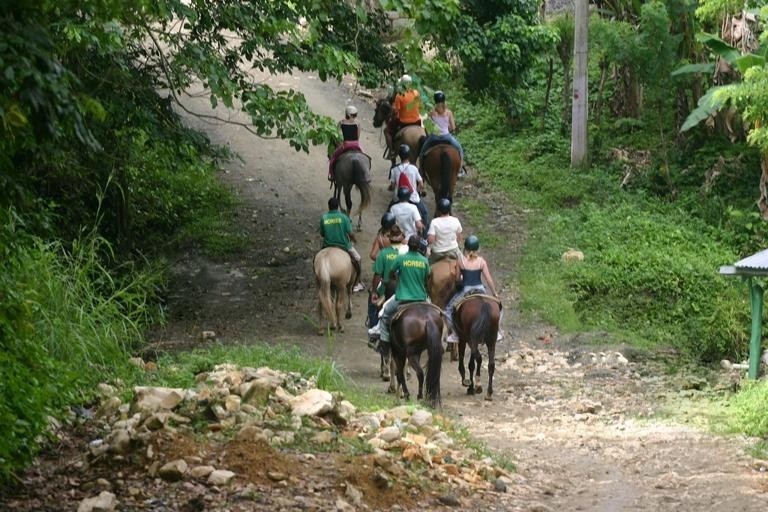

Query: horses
372,98,426,167
313,233,360,335
457,294,500,396
385,272,444,402
328,137,373,217
368,288,411,381
419,133,461,214
429,256,464,309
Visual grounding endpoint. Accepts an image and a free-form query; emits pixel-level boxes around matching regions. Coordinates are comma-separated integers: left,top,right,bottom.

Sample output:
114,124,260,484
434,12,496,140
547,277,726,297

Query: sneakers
446,333,460,343
352,284,360,292
357,283,364,290
368,325,380,335
458,168,465,178
379,343,390,355
496,334,503,341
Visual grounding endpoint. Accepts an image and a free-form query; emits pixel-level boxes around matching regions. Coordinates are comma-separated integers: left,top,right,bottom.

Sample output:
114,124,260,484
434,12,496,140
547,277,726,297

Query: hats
408,235,420,247
387,225,405,242
397,75,413,86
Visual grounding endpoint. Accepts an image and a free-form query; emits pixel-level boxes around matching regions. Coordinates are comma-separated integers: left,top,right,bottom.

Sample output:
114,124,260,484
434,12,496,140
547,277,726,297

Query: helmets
465,234,479,250
398,144,410,156
420,237,429,253
434,91,445,103
380,213,397,226
439,198,451,210
345,105,358,117
398,186,410,198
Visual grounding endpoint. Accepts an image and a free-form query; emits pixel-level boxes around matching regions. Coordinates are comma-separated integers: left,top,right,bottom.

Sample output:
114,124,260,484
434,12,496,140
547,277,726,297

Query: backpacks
398,172,414,193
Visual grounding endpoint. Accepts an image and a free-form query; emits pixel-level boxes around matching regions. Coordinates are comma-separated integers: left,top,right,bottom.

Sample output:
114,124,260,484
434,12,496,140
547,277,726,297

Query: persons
390,186,423,237
371,226,407,305
371,213,405,261
327,106,362,180
319,197,363,292
386,144,427,223
427,199,463,263
444,235,503,344
419,91,463,177
376,236,430,353
367,238,428,333
385,74,420,158
